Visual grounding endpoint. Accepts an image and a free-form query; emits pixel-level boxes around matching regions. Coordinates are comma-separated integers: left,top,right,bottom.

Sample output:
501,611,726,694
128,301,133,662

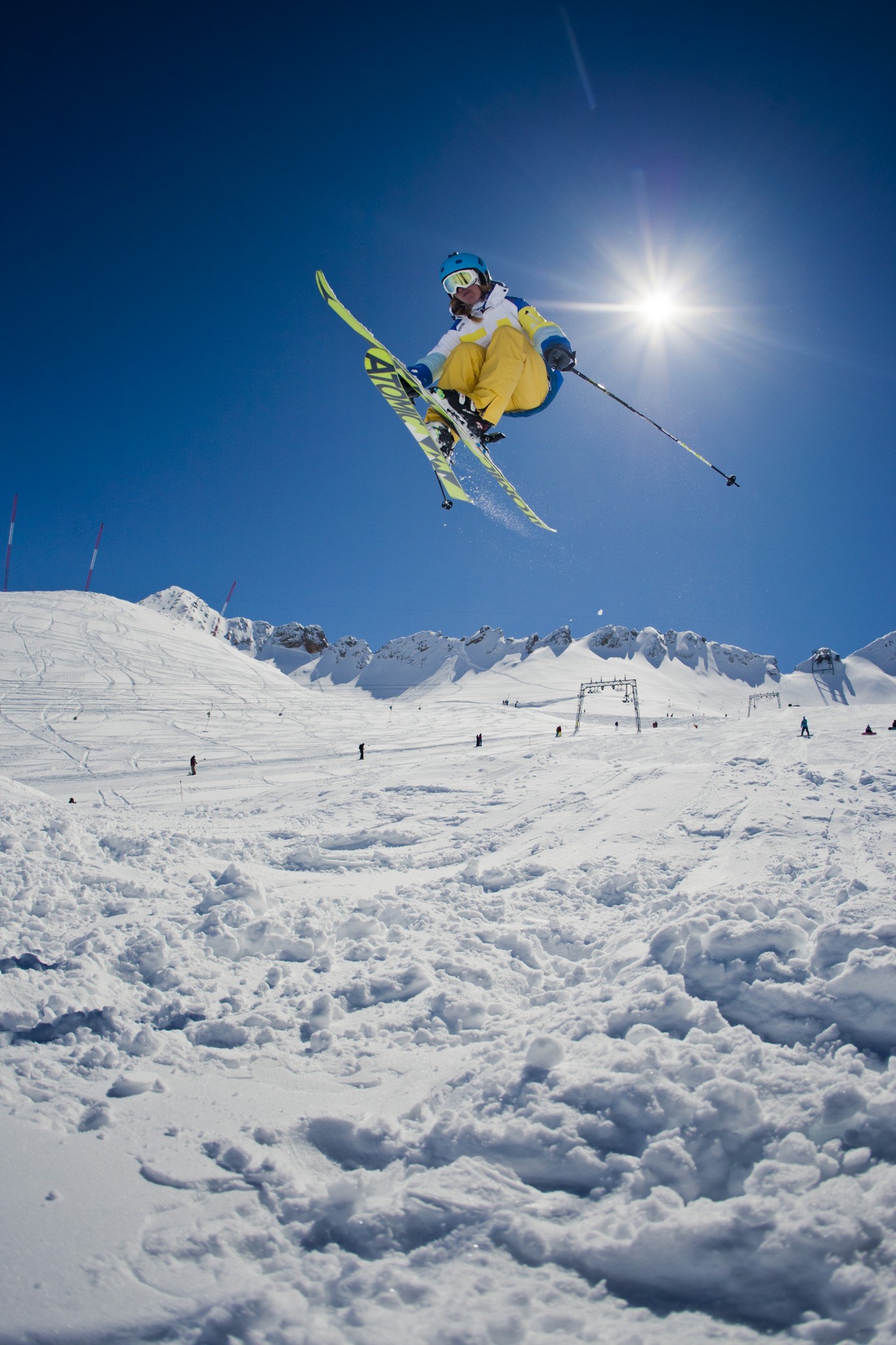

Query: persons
503,700,504,704
653,722,655,728
191,755,197,775
478,733,482,746
725,714,727,717
801,717,810,736
476,735,479,746
655,721,657,728
398,252,577,466
558,726,561,737
866,724,872,733
359,743,365,760
667,713,668,717
615,722,618,731
556,727,559,737
504,700,509,705
515,703,517,707
672,713,673,716
893,720,896,728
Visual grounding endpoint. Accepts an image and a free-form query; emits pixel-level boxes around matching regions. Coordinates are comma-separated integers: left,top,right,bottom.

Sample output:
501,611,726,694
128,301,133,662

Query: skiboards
315,268,558,533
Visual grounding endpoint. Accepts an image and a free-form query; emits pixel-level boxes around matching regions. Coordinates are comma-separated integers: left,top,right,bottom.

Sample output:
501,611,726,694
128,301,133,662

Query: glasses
442,269,478,294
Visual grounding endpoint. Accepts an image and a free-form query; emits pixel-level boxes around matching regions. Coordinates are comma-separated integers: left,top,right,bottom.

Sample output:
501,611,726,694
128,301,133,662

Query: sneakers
425,420,454,464
429,384,492,436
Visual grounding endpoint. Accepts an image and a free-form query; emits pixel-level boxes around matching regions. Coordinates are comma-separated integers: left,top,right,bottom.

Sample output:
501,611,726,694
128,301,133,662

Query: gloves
547,347,574,370
399,365,426,401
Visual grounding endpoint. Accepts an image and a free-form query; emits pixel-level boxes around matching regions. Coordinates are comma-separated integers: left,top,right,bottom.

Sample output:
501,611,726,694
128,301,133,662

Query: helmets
440,252,491,299
